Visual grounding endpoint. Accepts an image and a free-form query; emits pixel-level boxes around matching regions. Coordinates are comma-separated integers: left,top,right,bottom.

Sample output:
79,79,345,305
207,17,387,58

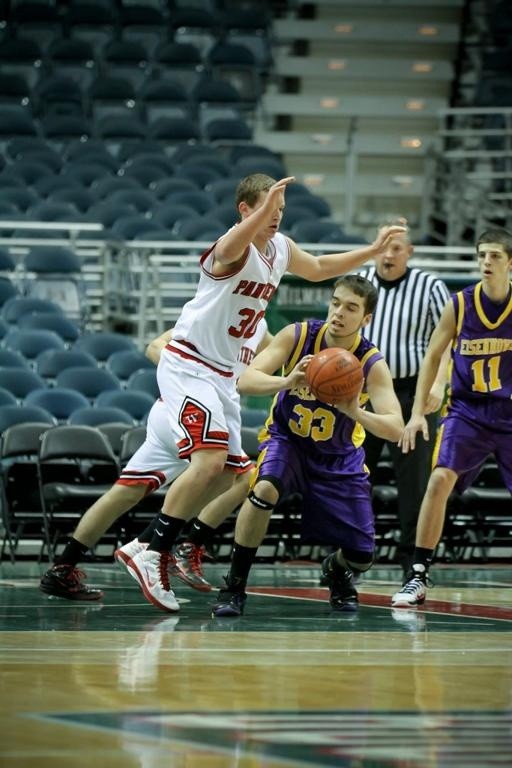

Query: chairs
1,242,512,569
0,3,368,241
432,0,511,242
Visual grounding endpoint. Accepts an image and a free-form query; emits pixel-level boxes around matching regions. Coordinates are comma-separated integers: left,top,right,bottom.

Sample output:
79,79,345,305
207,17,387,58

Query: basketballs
305,348,363,405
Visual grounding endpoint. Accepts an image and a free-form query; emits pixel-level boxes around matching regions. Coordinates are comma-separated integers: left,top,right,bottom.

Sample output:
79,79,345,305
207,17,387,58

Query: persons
210,271,407,620
389,229,512,609
36,310,280,604
343,218,453,585
113,171,403,613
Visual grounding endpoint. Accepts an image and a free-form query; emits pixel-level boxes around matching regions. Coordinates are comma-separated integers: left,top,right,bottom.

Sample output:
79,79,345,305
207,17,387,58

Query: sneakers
322,550,359,611
390,576,426,608
127,550,182,612
39,565,104,602
165,538,213,592
215,583,247,616
115,539,150,568
